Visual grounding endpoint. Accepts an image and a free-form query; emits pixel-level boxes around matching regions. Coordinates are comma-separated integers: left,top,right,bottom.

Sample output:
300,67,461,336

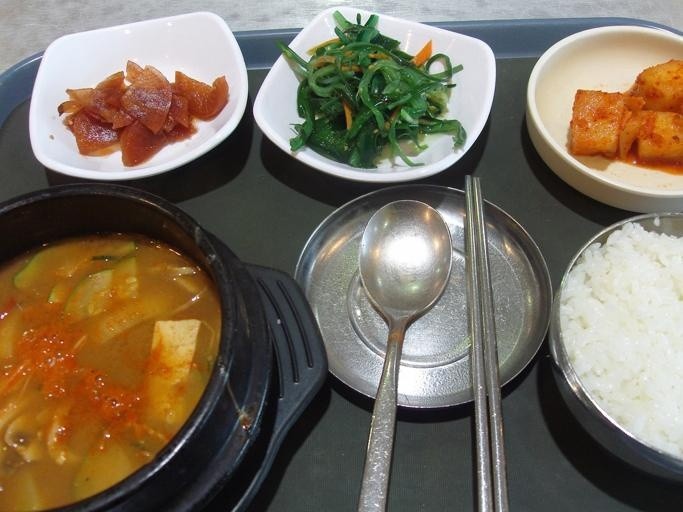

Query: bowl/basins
551,212,683,482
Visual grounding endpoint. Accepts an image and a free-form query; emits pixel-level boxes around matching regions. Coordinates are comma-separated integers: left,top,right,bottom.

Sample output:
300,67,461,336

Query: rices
561,217,682,464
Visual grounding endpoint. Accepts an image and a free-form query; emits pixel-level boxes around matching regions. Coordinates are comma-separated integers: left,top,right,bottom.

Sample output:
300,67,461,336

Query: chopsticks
463,174,510,507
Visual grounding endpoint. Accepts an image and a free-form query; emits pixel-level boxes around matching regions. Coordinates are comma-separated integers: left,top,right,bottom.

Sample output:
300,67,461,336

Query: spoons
357,200,454,507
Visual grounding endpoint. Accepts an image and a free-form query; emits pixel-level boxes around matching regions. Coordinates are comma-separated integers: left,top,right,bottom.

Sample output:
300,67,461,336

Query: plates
29,10,250,181
520,23,682,212
292,182,553,411
253,3,496,180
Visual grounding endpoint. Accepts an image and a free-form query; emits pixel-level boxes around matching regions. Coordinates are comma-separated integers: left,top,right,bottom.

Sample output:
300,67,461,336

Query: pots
0,181,327,512
